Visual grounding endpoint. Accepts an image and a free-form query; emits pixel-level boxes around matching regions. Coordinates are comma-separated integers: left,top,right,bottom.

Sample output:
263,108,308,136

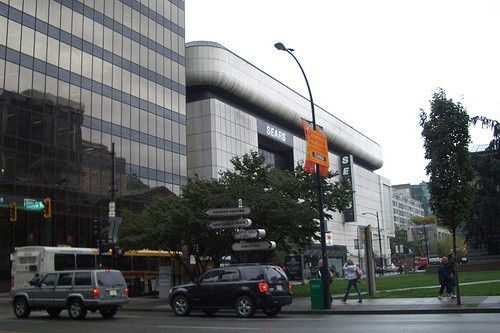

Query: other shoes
447,293,456,298
358,300,363,303
340,298,345,304
437,295,442,299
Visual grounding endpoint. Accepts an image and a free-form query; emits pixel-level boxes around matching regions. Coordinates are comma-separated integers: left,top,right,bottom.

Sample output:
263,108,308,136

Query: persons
438,257,457,300
340,259,362,304
448,249,456,296
31,273,40,282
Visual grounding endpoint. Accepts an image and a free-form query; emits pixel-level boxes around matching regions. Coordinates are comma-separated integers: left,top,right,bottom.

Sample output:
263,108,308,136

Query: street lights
84,142,116,270
362,211,384,276
274,41,331,310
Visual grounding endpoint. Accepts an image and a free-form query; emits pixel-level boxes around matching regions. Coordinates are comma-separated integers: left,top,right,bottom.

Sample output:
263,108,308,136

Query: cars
418,258,428,267
429,255,440,265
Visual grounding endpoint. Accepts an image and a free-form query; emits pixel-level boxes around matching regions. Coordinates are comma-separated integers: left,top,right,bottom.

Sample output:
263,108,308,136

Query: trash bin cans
310,279,325,309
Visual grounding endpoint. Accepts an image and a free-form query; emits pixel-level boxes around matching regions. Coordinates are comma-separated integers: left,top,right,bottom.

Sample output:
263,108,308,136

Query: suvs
167,262,293,318
9,269,130,320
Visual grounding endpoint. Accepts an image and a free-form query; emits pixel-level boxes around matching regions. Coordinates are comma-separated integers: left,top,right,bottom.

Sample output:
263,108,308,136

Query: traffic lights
42,198,51,219
9,202,17,222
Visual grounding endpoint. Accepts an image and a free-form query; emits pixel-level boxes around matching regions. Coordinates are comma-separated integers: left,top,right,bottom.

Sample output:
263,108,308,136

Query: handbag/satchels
355,267,363,279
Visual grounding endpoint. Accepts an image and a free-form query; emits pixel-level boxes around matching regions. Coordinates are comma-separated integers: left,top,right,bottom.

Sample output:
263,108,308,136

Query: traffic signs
233,228,266,240
231,240,276,252
206,218,252,230
203,207,251,218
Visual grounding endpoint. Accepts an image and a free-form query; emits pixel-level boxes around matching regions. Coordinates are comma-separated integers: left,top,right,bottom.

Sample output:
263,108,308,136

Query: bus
9,243,184,304
